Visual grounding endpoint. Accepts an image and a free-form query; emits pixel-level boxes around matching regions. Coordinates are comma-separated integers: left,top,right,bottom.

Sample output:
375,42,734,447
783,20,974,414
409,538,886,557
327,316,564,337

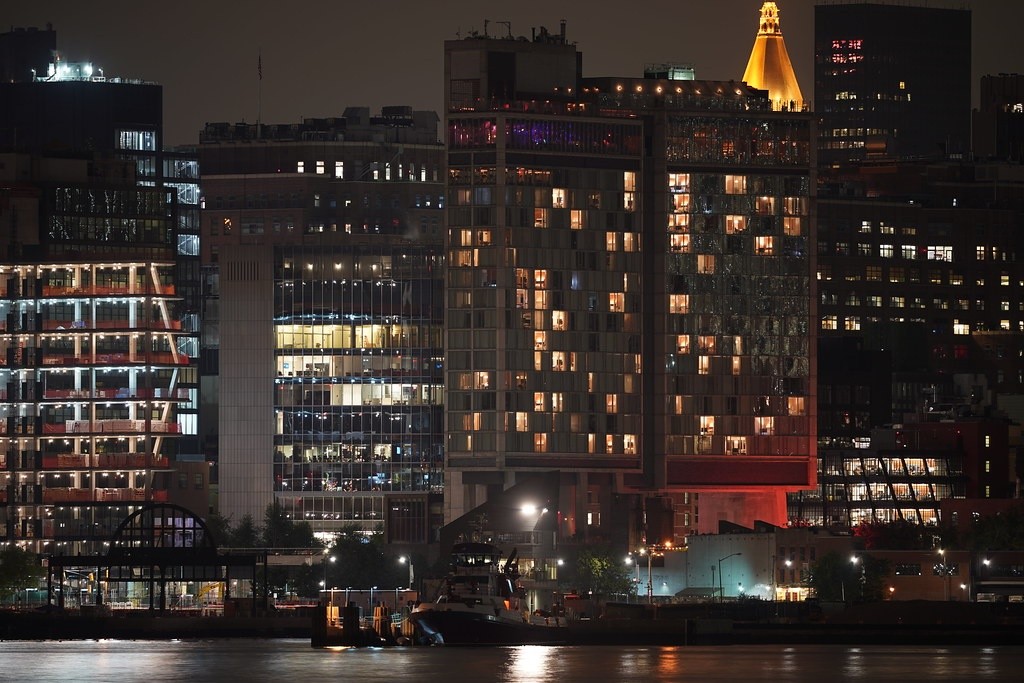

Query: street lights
345,586,353,607
936,547,947,601
330,586,337,607
718,553,743,603
395,587,403,613
369,585,379,618
324,552,337,589
397,552,413,589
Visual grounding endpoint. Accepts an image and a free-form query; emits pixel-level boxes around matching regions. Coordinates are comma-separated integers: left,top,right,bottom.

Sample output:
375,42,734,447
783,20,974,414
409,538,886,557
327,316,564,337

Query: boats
410,541,585,647
